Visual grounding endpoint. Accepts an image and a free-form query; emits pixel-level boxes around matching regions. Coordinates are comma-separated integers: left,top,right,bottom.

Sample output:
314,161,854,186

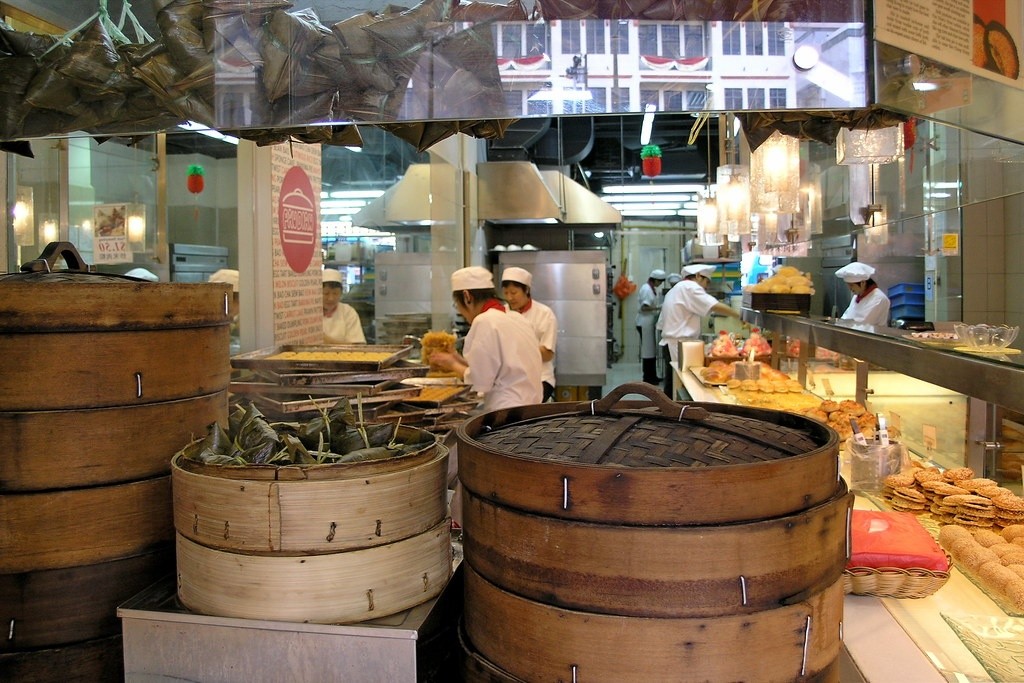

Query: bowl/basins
953,322,1020,353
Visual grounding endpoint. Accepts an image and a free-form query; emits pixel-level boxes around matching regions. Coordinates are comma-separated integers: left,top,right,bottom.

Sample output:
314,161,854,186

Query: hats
321,268,343,283
681,264,718,281
207,268,239,292
834,262,877,283
450,266,496,291
124,267,159,282
501,266,534,286
667,273,682,282
649,269,668,280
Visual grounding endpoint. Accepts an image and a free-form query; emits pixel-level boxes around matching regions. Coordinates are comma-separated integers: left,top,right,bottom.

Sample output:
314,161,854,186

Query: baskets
841,534,954,600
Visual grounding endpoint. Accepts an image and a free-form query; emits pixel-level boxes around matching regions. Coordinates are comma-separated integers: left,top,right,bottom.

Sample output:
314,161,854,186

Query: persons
835,261,891,328
428,264,544,412
499,266,560,403
658,264,754,400
635,269,666,385
667,274,682,289
208,269,240,334
322,269,368,345
125,267,159,282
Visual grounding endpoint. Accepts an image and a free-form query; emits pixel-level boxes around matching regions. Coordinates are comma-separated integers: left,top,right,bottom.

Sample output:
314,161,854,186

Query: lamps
39,143,60,253
697,114,905,245
126,139,148,243
13,183,36,245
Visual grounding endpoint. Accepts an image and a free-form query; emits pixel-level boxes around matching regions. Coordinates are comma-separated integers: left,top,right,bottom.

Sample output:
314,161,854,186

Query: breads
751,265,816,295
881,468,1024,616
785,400,901,450
699,360,804,394
712,333,774,357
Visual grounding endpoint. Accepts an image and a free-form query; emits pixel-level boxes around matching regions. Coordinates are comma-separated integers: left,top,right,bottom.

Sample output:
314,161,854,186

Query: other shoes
642,377,663,386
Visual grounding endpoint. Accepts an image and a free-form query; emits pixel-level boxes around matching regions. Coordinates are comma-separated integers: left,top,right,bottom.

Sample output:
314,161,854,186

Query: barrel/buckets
735,362,760,381
850,438,901,492
